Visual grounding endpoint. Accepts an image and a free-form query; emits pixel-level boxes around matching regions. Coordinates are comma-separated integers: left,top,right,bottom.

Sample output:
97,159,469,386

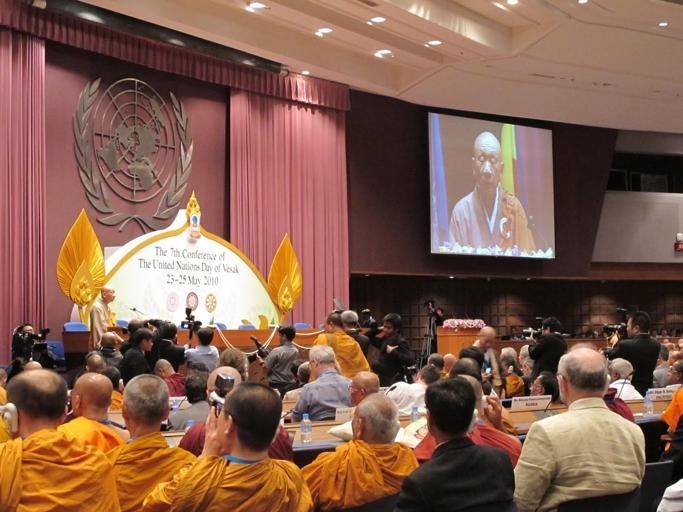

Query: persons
500,348,516,363
291,345,352,420
427,302,446,353
443,353,456,377
152,359,185,396
122,329,152,388
11,324,34,361
666,360,683,386
661,328,670,337
501,355,523,379
56,372,130,455
100,332,122,367
393,376,514,512
104,374,197,512
412,374,522,469
185,328,219,371
383,366,440,409
363,313,415,387
340,311,369,359
310,313,369,380
217,347,246,381
650,329,659,338
450,131,536,252
91,286,116,346
519,344,535,370
142,381,313,512
607,358,644,400
611,312,661,397
119,319,144,353
473,326,495,367
448,358,482,383
529,318,569,387
282,361,310,400
166,374,209,431
299,393,418,510
652,346,669,389
428,354,444,375
459,346,484,368
176,366,294,464
85,355,105,373
348,370,379,407
514,348,645,512
257,325,298,398
146,324,185,373
100,366,122,413
530,371,559,404
0,369,121,512
0,367,7,403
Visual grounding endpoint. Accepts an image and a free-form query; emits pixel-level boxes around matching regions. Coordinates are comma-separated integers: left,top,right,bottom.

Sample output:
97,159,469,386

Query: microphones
618,369,637,398
532,390,559,420
125,305,146,320
387,384,399,396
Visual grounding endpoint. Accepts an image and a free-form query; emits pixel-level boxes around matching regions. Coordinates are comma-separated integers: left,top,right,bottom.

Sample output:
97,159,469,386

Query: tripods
420,313,437,374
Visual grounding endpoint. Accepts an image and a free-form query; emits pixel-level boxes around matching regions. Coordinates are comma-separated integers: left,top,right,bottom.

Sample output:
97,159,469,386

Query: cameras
333,298,346,314
250,335,269,359
424,300,435,309
11,328,50,351
523,317,543,339
604,321,628,338
180,307,202,332
207,373,234,418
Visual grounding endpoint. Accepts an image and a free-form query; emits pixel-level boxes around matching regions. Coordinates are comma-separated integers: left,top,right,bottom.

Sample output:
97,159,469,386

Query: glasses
529,382,543,387
347,385,367,392
309,360,319,370
667,366,680,373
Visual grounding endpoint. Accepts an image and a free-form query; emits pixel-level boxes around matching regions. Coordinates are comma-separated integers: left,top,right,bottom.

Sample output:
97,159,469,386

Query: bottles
411,405,420,424
300,414,312,443
185,420,194,434
643,392,653,417
468,409,478,435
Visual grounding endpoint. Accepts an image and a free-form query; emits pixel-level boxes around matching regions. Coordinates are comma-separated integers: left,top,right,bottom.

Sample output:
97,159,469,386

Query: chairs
239,326,257,329
502,400,515,409
319,416,336,422
211,323,226,329
319,323,326,328
63,323,90,331
634,419,667,455
176,326,187,328
515,434,530,444
294,323,311,329
557,486,639,512
160,422,167,433
116,320,129,326
293,446,334,467
641,461,673,512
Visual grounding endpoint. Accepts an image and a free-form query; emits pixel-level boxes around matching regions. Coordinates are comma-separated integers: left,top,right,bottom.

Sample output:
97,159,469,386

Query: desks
434,324,683,359
163,399,671,448
63,328,365,358
107,400,298,446
